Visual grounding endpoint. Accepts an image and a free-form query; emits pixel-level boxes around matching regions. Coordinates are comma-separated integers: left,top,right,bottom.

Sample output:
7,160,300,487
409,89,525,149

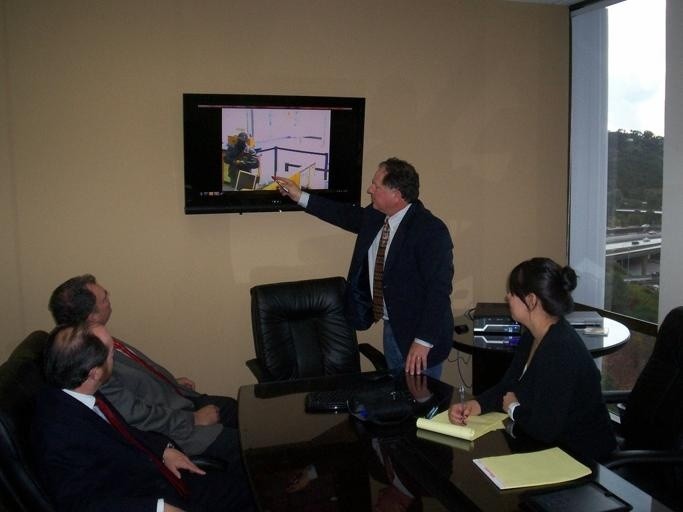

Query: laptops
564,310,603,326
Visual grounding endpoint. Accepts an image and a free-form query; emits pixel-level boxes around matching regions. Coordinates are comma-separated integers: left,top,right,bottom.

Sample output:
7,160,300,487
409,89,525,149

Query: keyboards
306,390,417,413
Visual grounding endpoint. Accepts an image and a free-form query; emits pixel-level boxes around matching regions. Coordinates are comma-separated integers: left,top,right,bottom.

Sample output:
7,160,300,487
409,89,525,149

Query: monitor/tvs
183,94,365,214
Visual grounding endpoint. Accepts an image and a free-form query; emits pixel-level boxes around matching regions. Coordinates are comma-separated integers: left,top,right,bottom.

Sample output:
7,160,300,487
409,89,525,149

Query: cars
643,237,650,242
648,231,656,235
641,224,650,227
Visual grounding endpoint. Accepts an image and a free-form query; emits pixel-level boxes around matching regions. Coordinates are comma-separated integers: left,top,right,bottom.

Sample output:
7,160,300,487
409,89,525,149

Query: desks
453,315,631,396
235,367,675,512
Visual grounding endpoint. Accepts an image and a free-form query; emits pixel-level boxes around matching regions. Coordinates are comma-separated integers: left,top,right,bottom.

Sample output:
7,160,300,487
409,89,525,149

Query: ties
93,396,194,503
373,221,390,325
112,340,185,400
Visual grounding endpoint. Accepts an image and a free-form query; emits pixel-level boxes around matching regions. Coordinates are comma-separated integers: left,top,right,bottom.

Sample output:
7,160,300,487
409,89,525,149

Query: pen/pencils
460,385,464,423
271,176,289,193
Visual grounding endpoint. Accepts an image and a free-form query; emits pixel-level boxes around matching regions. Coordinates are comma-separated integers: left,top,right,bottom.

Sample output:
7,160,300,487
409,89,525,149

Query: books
415,406,509,441
471,447,594,491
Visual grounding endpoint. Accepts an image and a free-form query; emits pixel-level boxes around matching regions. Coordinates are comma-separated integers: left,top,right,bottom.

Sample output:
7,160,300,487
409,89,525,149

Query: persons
273,156,455,381
48,273,244,467
30,320,257,512
348,368,456,512
222,132,259,192
445,255,621,465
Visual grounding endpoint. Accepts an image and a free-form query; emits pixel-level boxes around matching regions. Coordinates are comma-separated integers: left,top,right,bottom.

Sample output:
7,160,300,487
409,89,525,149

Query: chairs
0,330,71,511
246,274,388,385
603,306,683,512
